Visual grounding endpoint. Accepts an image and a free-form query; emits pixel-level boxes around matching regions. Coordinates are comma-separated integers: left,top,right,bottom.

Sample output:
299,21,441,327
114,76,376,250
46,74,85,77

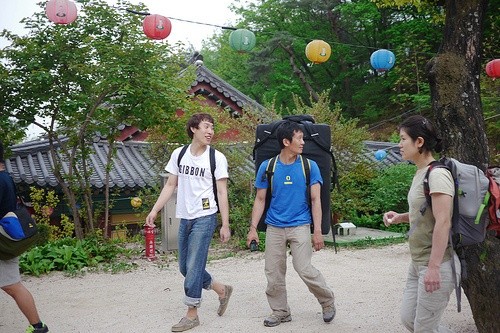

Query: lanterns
230,28,256,52
131,197,142,208
369,49,395,76
46,0,76,25
305,39,331,64
143,15,172,39
485,59,500,78
375,151,387,161
41,206,53,221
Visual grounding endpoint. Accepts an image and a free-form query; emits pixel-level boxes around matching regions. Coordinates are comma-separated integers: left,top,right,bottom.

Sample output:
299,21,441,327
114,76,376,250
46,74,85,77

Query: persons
146,113,232,332
383,115,462,333
0,146,48,333
246,124,336,327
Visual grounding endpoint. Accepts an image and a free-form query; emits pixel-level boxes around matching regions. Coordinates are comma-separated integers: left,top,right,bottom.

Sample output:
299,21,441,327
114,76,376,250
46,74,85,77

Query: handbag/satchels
0,206,38,260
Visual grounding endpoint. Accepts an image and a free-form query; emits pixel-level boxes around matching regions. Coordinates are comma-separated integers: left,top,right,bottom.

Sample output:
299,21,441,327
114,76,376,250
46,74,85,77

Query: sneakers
323,301,335,322
264,314,292,327
25,322,48,333
172,315,199,331
217,284,232,316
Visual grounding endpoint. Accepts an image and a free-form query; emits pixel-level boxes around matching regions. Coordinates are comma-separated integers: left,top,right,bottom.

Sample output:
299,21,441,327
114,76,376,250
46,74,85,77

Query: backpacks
423,155,500,249
253,115,332,234
